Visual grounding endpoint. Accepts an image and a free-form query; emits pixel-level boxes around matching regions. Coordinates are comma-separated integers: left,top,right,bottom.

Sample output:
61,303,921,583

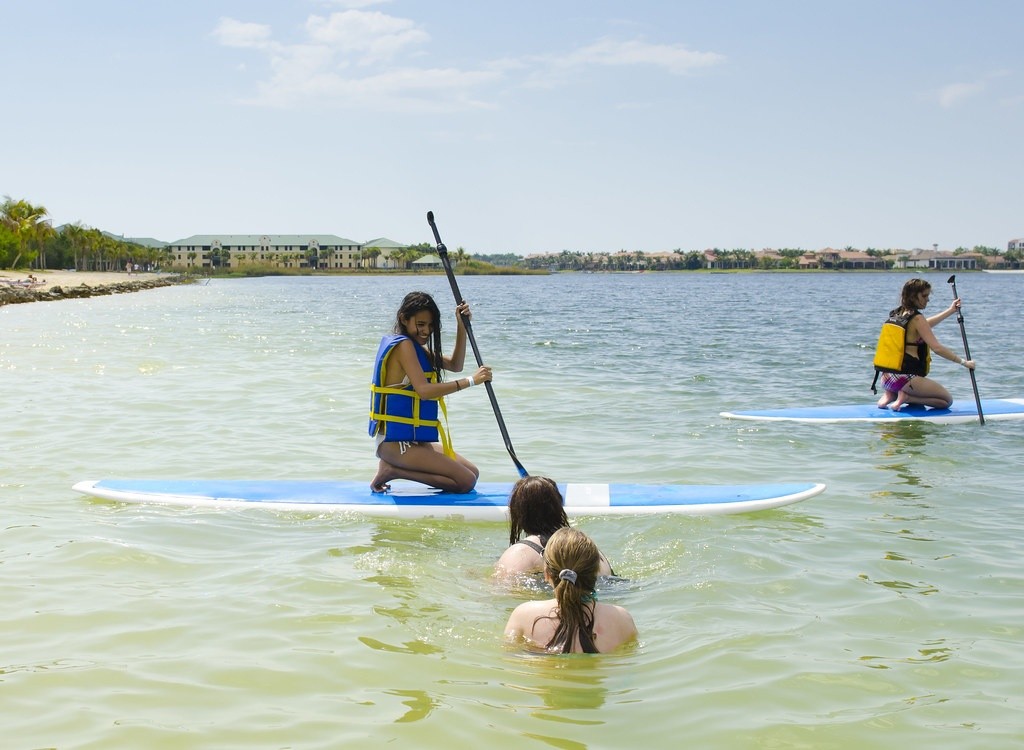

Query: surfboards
716,397,1023,425
69,475,827,521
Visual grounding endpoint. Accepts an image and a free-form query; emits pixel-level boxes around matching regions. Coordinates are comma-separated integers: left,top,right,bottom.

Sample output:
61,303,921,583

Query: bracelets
455,380,461,390
467,376,474,386
960,359,965,365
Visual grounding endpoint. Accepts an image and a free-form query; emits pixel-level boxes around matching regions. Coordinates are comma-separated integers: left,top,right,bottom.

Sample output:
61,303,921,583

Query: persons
507,528,636,653
369,292,492,493
871,278,976,411
499,476,612,576
126,261,139,276
18,274,34,283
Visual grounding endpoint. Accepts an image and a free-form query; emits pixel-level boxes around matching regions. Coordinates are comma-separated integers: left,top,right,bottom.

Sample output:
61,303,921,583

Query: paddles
426,209,532,478
946,273,987,426
